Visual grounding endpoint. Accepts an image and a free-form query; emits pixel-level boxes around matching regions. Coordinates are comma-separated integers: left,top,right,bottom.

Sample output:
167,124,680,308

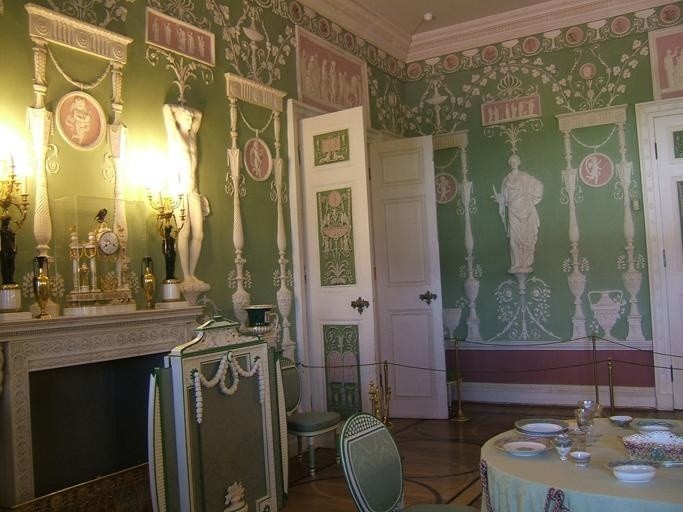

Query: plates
606,413,683,485
495,416,569,461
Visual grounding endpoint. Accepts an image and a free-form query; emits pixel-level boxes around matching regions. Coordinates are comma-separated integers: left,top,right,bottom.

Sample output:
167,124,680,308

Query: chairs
279,355,480,512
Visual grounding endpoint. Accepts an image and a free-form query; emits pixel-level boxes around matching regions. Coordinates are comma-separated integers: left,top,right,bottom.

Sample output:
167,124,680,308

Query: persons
162,100,213,295
493,152,544,275
185,31,195,57
299,50,366,117
151,17,160,43
164,224,178,281
195,34,206,59
0,213,17,285
174,25,187,52
161,21,174,45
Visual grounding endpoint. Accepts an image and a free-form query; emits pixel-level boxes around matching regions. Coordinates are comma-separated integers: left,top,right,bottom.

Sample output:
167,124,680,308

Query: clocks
96,221,120,263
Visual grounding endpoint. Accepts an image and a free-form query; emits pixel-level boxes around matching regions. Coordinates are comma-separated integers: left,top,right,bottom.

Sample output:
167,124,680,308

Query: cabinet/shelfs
0,304,207,512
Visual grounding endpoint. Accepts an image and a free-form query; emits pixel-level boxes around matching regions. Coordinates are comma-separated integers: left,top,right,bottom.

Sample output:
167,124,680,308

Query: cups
242,304,279,328
553,396,602,466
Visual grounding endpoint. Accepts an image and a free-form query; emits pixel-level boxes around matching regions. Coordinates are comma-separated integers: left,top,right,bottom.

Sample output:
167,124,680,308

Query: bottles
138,255,157,310
31,257,53,321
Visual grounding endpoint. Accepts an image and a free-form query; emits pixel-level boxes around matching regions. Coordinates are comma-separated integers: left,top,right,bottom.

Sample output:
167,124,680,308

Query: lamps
0,144,28,316
143,155,190,310
